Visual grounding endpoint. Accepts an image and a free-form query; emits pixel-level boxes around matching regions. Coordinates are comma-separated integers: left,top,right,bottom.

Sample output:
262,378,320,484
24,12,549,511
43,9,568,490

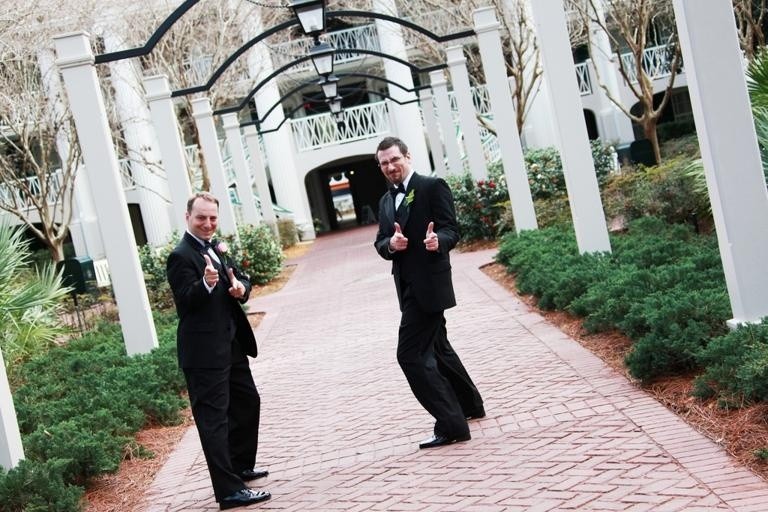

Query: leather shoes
219,488,272,509
418,425,473,450
464,405,488,420
237,467,269,483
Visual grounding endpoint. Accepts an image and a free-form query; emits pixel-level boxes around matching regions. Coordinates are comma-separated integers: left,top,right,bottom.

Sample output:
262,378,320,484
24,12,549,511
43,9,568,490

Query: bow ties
202,238,218,252
389,180,406,198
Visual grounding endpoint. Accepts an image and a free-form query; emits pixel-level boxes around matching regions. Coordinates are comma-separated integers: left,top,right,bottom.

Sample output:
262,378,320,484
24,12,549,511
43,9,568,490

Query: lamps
286,1,345,123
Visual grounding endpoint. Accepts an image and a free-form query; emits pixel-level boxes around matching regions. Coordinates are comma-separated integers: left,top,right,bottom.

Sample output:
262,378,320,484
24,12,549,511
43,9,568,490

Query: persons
374,135,486,450
165,191,273,511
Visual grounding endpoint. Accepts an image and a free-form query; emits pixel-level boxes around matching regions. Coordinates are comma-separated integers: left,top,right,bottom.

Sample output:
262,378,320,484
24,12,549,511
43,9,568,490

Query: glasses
376,154,406,168
